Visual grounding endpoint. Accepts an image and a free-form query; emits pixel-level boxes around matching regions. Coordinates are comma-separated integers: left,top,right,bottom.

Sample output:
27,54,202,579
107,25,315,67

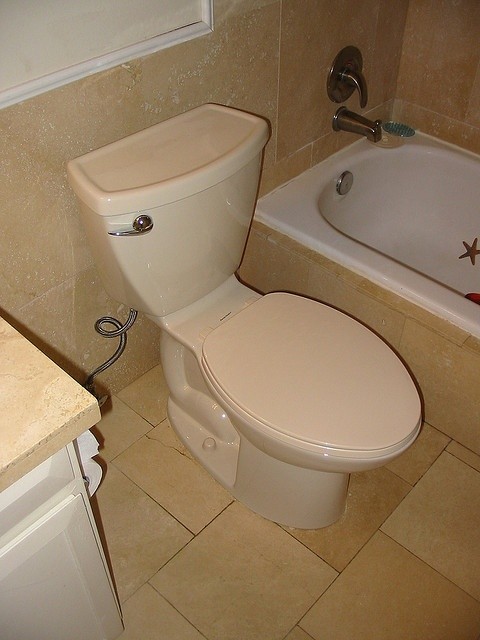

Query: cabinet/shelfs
1,440,126,639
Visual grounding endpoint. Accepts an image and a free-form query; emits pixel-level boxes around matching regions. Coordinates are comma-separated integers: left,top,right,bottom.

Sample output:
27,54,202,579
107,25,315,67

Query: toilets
67,102,424,532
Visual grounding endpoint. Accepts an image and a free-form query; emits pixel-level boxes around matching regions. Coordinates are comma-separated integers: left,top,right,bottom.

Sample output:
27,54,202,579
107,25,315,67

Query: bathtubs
254,124,480,338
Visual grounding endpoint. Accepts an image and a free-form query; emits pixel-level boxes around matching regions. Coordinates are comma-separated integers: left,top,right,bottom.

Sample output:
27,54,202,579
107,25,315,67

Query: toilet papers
72,430,102,500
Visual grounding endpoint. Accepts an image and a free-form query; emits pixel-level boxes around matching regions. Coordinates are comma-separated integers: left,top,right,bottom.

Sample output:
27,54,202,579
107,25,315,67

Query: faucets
332,105,385,143
